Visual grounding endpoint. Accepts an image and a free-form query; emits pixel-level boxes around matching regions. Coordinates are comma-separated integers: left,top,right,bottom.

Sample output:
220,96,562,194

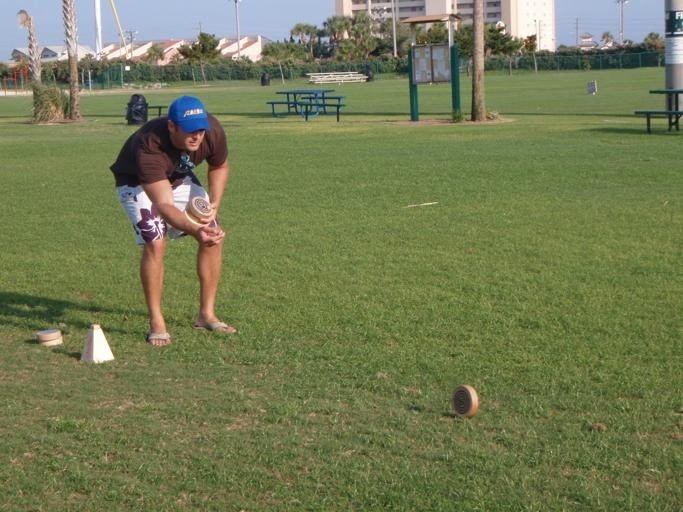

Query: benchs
266,95,346,122
633,109,673,134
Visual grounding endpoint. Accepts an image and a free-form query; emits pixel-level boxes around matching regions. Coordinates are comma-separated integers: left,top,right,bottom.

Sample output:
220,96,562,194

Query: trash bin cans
261,72,271,86
126,94,148,125
366,71,374,82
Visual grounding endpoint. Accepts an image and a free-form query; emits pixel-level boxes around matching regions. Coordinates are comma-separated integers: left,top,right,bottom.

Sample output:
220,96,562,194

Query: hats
168,95,210,132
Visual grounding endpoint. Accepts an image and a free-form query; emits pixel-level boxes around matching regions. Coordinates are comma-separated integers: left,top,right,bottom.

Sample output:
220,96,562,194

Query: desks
276,88,335,114
649,86,683,132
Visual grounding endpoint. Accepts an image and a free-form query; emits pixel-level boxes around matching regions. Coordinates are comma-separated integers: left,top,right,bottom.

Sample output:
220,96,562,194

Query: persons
109,95,237,347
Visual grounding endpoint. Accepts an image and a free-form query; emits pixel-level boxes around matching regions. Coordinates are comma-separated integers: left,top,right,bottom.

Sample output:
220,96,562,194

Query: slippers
194,321,237,334
147,333,171,346
